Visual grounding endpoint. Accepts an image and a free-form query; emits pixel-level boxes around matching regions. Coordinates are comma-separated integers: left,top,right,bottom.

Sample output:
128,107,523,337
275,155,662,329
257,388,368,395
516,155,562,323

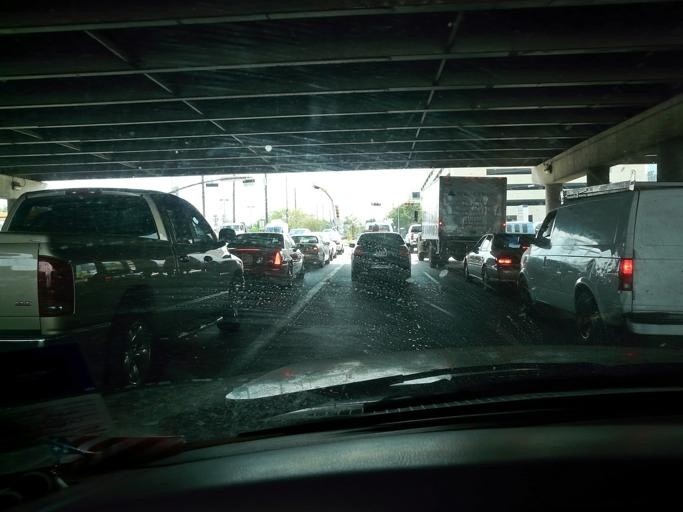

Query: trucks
414,176,510,269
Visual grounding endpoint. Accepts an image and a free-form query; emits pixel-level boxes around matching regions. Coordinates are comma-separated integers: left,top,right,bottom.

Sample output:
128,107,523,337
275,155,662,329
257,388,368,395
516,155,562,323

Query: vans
516,183,682,345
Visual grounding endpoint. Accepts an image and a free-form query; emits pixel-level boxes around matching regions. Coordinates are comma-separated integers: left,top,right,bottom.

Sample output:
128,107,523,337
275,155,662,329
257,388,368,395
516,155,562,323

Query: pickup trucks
0,189,245,387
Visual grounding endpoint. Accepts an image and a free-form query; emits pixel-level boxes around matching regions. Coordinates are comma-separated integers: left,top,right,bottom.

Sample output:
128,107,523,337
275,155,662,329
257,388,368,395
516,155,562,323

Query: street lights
311,183,336,230
